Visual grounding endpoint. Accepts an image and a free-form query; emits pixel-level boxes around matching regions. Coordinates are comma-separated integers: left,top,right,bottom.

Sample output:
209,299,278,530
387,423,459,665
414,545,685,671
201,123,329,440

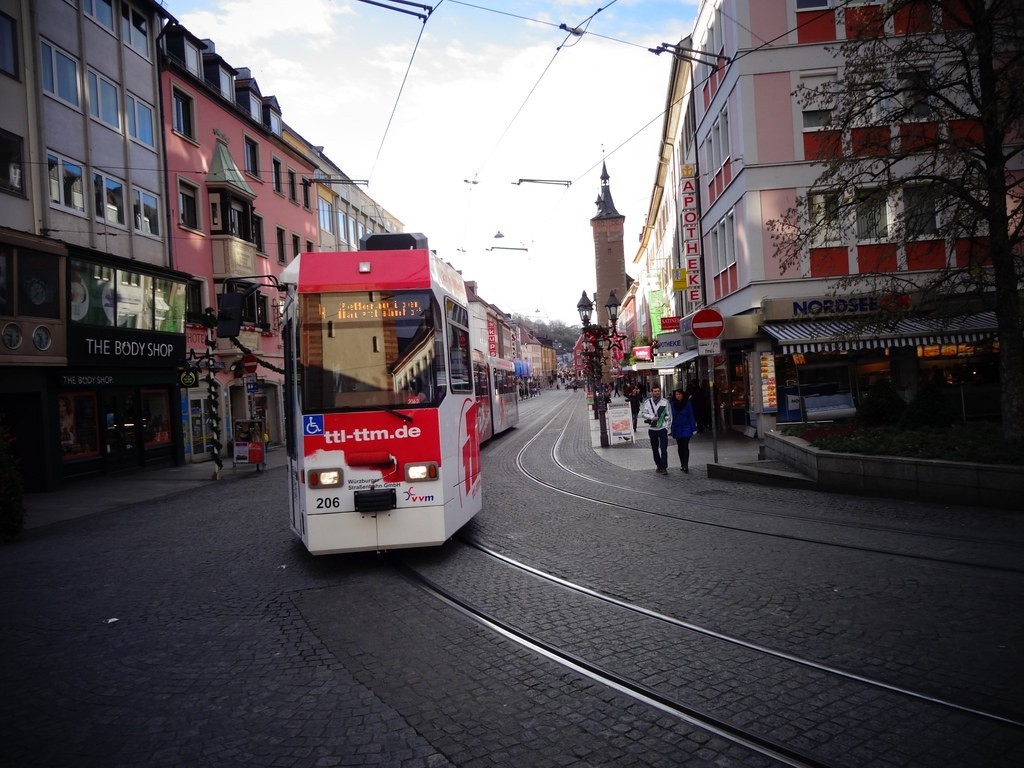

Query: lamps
494,230,505,238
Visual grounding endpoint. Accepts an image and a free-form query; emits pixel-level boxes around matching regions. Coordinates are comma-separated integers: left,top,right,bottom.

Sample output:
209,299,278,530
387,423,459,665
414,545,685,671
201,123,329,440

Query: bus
217,233,519,556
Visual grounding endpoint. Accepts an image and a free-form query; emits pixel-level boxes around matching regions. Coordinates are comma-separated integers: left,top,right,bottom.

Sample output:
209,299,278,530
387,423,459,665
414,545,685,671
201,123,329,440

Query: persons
625,389,643,432
394,357,430,402
59,397,77,448
600,382,648,409
664,389,698,473
684,377,721,434
641,386,673,475
556,372,578,391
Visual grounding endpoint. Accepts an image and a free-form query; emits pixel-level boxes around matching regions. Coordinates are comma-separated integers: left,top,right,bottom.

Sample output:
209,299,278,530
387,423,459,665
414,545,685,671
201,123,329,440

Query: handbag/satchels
644,398,657,426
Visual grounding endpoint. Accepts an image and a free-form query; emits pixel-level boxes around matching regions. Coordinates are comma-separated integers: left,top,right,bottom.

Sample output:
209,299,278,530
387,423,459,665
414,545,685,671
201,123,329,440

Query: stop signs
244,354,257,373
692,309,724,340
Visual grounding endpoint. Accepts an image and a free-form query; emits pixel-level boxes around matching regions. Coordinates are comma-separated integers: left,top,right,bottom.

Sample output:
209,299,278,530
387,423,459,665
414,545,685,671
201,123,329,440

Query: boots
678,449,684,471
684,449,689,473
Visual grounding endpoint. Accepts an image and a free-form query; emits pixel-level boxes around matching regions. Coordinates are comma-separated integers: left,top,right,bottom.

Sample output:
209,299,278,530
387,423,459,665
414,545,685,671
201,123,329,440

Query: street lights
576,290,622,448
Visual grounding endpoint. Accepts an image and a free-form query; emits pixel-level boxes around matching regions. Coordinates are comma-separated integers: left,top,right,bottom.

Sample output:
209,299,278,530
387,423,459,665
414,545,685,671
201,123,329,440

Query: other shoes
660,467,668,475
656,467,662,473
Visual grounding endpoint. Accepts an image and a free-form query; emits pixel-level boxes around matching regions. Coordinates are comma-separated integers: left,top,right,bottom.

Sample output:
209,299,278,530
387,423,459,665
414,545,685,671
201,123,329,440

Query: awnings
760,310,999,355
652,348,699,369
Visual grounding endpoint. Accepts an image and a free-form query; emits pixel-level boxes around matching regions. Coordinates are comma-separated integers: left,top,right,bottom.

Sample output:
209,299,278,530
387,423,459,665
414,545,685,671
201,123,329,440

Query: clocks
33,323,53,351
2,321,26,350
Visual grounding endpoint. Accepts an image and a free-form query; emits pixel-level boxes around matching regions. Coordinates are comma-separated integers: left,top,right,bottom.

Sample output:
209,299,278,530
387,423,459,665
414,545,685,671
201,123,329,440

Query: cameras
644,419,652,425
